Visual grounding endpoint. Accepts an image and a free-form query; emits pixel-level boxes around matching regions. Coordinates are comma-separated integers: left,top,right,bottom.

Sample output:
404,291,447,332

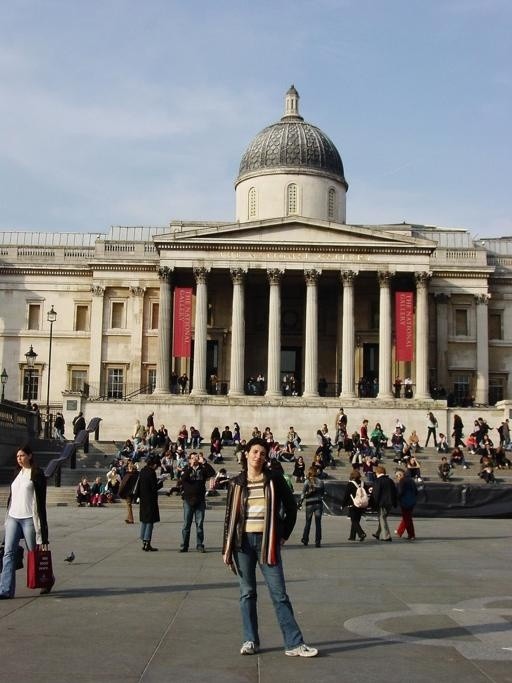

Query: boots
143,541,157,551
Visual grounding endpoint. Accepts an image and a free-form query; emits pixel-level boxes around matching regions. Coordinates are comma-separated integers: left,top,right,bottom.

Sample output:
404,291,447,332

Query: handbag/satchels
27,551,52,589
0,546,24,573
132,480,140,495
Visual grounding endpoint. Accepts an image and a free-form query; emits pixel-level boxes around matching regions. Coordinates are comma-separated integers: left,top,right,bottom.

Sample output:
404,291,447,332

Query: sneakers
441,478,450,482
285,644,319,657
40,576,55,594
198,548,204,553
241,641,259,654
301,538,308,545
125,520,134,523
360,533,366,541
316,543,320,547
395,530,401,537
372,534,392,541
418,478,422,482
177,548,188,552
348,538,355,541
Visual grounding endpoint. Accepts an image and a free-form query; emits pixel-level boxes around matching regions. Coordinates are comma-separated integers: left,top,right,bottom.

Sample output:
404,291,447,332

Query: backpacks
349,480,368,508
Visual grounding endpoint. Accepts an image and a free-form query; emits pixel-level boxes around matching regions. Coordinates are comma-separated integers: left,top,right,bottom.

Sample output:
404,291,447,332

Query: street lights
0,367,10,399
24,345,38,409
44,302,59,441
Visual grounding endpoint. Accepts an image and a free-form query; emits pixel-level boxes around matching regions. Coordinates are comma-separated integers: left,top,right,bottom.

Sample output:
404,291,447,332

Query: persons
173,372,217,394
33,404,86,438
315,409,512,484
248,375,298,397
0,447,56,598
210,422,305,488
78,412,216,553
300,466,418,547
429,383,474,408
222,439,318,657
358,375,413,399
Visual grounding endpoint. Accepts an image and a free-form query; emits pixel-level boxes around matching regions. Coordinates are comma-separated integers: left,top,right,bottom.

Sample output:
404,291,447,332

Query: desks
322,480,512,518
